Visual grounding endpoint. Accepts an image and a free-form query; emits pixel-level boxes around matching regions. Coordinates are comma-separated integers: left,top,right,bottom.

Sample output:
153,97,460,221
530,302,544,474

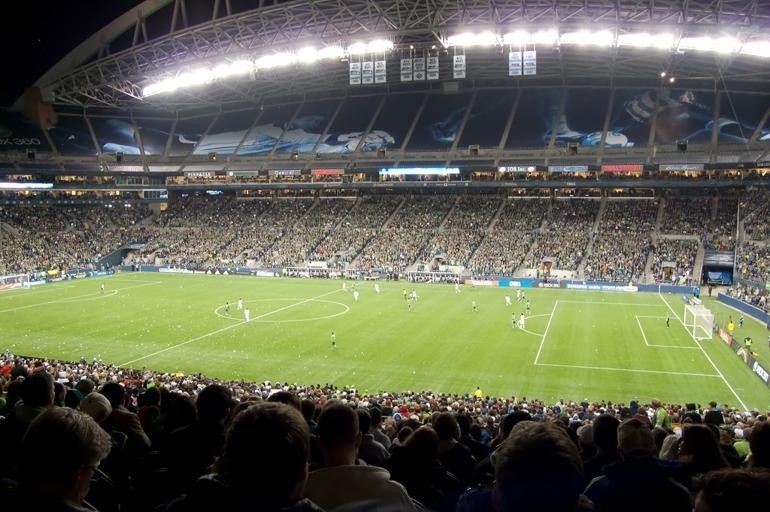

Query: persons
471,298,480,313
1,172,770,282
744,335,753,351
666,312,670,328
728,320,735,338
587,84,769,146
670,282,770,315
44,107,385,160
330,329,337,348
412,289,418,301
738,313,744,327
504,287,532,329
350,282,356,292
454,282,462,295
374,282,381,295
354,290,359,303
237,298,244,311
100,282,106,294
408,292,413,299
244,308,251,323
2,348,770,512
428,83,583,148
223,302,232,318
355,282,360,291
406,298,413,311
342,281,348,294
400,288,408,301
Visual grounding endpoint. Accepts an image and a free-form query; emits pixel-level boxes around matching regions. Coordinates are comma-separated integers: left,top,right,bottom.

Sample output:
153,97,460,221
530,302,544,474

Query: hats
576,424,594,443
80,392,112,422
